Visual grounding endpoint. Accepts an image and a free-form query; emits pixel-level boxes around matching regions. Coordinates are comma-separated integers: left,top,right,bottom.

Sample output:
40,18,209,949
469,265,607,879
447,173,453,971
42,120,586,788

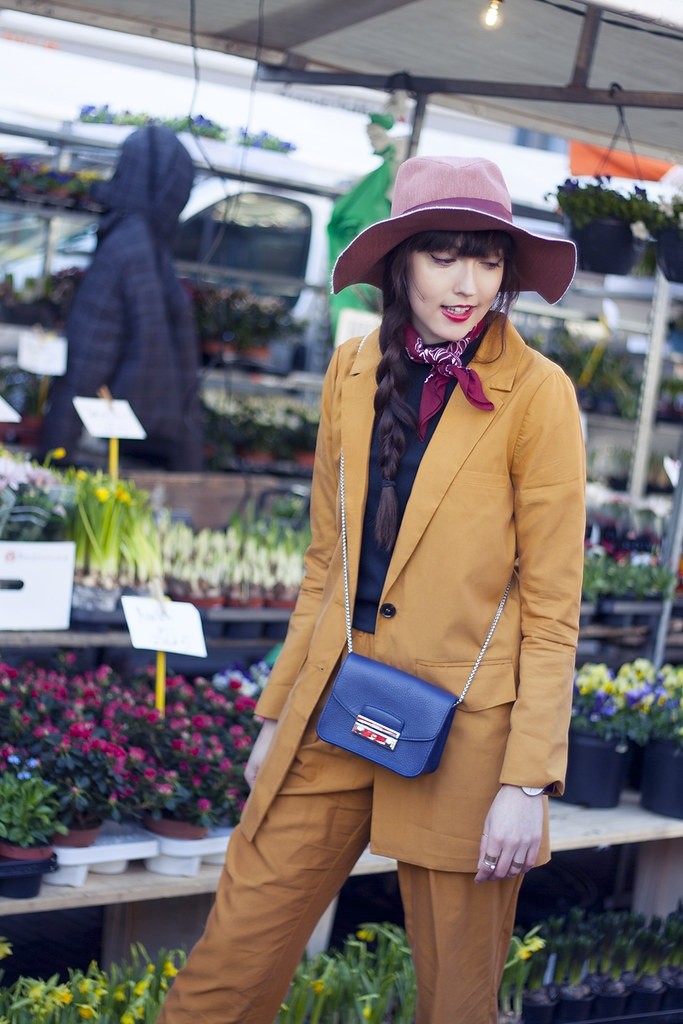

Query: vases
12,416,317,472
573,216,635,274
75,121,290,174
0,298,276,362
549,725,683,822
1,540,296,638
0,175,105,212
0,814,234,902
653,229,683,283
586,413,683,482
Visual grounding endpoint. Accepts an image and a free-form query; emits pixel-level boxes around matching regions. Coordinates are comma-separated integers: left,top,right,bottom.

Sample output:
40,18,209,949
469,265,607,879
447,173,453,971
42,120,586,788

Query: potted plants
514,902,683,1024
577,550,673,630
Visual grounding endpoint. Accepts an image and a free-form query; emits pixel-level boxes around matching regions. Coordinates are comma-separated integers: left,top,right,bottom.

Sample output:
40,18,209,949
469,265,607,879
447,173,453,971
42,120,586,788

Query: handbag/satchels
315,653,459,778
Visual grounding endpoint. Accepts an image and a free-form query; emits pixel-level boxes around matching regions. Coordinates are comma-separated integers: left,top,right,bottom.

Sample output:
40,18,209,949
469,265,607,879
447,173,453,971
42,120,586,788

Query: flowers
0,258,306,329
0,916,546,1024
552,172,651,225
630,186,683,233
533,340,683,427
0,372,327,446
83,101,293,154
0,153,114,201
570,655,683,738
1,463,312,596
0,657,275,840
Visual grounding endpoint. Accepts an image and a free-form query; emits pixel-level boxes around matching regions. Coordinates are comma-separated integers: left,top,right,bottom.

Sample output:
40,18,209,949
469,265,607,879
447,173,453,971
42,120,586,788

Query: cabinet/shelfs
0,261,683,959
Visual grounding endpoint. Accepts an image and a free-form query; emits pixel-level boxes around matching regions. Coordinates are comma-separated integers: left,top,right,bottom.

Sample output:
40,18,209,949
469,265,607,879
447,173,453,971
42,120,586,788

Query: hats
333,156,577,306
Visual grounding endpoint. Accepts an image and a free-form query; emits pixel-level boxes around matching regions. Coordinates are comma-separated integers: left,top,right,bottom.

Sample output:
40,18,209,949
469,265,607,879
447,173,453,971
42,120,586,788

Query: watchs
521,783,554,797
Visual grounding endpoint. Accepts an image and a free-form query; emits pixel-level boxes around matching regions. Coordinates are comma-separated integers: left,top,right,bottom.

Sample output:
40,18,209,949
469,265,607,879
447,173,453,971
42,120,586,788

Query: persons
155,157,586,1024
35,127,199,471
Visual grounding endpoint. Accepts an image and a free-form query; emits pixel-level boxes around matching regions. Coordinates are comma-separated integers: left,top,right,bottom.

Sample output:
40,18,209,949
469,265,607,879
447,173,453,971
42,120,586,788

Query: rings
485,861,496,869
484,853,499,863
511,861,523,868
